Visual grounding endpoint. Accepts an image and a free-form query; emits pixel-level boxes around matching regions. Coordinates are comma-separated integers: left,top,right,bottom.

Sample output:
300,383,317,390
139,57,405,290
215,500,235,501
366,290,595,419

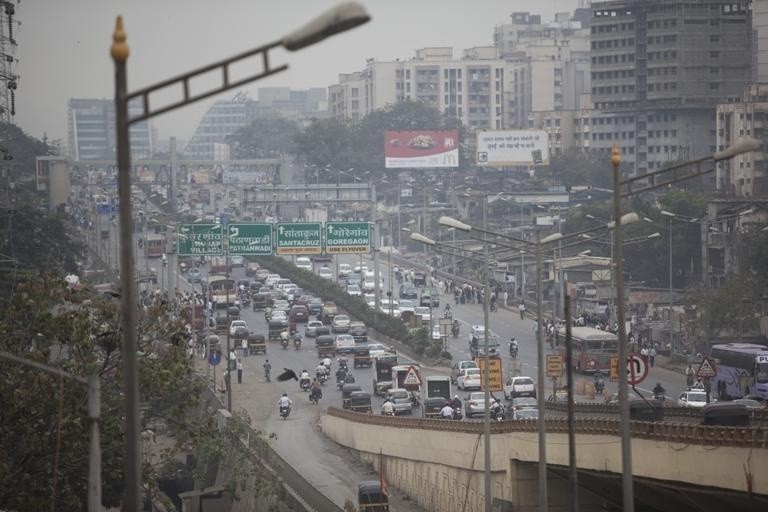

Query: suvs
701,402,752,435
628,399,664,422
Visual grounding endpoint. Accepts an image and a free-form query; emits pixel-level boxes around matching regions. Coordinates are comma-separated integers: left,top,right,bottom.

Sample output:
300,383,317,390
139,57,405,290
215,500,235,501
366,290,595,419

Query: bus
555,324,618,377
706,338,768,401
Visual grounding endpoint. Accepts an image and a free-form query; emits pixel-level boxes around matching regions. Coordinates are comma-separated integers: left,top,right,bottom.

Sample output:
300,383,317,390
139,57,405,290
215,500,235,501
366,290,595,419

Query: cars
732,395,764,409
675,387,717,407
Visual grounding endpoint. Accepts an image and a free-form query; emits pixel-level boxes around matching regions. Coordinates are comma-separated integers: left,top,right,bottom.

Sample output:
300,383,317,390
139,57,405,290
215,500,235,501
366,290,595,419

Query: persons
266,307,330,350
509,337,519,352
440,395,462,420
277,393,292,416
380,394,396,416
439,278,508,313
575,312,619,335
394,264,436,287
237,283,253,307
594,375,610,401
226,336,272,382
534,319,563,349
452,320,459,338
653,382,666,400
152,287,216,360
302,356,353,403
628,332,671,367
520,302,525,319
679,364,700,398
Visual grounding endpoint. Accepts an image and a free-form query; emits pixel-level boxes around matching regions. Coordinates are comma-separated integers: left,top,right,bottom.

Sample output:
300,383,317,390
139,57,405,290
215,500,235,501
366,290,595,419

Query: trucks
358,480,390,512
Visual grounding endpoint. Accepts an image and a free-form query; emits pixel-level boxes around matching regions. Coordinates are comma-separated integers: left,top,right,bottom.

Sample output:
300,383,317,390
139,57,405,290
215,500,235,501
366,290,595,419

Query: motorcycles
686,373,694,386
592,379,605,394
655,392,665,402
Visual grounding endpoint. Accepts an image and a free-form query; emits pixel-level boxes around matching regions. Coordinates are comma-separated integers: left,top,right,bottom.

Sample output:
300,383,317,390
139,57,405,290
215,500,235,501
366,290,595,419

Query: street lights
575,207,768,366
409,227,563,511
233,142,503,216
438,211,641,512
609,132,765,511
108,1,376,511
